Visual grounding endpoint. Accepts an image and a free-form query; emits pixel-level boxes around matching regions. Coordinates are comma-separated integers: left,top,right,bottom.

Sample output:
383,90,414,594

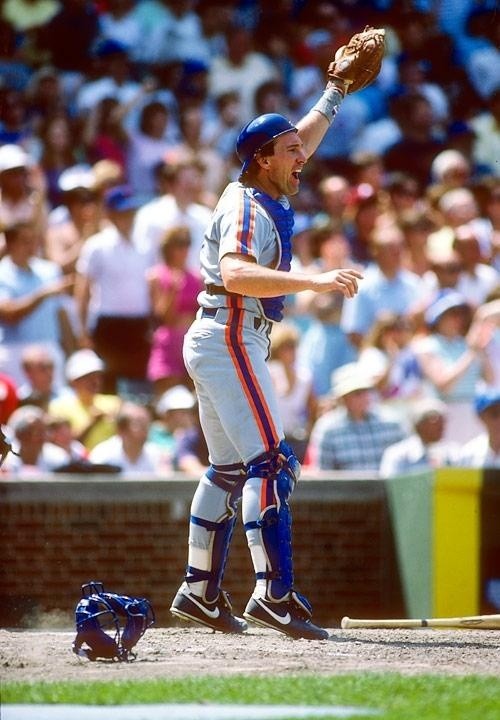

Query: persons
1,2,498,482
145,21,396,642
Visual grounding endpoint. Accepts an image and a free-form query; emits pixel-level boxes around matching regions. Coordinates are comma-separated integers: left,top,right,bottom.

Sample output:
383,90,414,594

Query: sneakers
169,581,248,634
243,593,328,641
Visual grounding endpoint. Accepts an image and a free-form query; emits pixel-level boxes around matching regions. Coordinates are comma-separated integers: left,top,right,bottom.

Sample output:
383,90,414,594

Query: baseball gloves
328,27,386,95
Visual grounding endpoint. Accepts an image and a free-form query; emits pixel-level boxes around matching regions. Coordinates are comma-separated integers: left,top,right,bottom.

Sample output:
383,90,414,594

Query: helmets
236,113,298,176
71,581,156,664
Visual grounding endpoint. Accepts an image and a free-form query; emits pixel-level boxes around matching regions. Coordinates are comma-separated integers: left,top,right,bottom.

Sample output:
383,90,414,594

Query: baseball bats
341,614,500,629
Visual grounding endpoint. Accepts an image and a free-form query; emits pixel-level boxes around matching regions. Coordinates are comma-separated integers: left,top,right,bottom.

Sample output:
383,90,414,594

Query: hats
103,185,142,212
63,348,106,382
476,391,500,414
330,360,376,399
156,384,196,417
0,143,29,173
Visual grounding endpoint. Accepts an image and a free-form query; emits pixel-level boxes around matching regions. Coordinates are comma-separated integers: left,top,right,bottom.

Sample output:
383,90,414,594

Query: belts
201,308,272,334
